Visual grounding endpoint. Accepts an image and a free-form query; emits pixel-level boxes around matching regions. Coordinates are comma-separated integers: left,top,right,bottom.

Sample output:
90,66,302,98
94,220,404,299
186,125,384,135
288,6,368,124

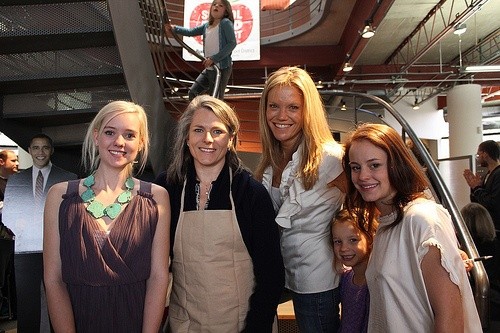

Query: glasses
476,151,483,158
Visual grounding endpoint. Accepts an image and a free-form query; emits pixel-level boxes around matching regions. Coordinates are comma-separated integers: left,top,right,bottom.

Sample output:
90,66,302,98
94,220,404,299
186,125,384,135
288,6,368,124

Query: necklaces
81,167,136,220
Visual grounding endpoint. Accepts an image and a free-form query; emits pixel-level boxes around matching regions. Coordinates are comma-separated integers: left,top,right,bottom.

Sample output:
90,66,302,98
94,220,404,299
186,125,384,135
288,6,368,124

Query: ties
34,170,45,210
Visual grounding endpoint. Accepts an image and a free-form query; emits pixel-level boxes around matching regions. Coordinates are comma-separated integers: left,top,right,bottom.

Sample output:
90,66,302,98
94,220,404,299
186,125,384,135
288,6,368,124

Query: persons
460,199,500,294
166,0,236,111
251,66,473,333
342,122,483,333
1,134,77,333
464,140,500,234
153,94,284,333
332,208,380,332
0,151,19,333
43,99,172,333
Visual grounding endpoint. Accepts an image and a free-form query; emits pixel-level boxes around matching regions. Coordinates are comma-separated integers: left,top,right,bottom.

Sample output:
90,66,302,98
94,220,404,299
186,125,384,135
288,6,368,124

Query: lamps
459,64,500,72
340,101,346,111
342,60,354,71
315,77,324,88
412,98,420,110
360,19,375,39
453,23,467,35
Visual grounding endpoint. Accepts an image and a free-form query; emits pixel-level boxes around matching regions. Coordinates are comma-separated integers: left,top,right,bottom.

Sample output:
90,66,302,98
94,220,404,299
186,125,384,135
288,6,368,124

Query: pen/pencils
463,256,493,263
